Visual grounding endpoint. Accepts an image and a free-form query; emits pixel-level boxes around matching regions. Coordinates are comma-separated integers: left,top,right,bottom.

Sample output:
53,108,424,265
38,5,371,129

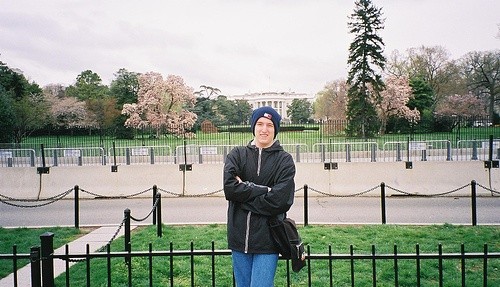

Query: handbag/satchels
270,218,306,272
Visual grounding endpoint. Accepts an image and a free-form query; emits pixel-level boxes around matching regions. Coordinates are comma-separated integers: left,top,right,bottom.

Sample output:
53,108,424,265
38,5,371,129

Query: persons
223,106,296,287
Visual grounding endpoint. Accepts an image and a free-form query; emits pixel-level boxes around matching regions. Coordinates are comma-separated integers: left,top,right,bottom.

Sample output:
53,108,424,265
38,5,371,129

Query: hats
251,107,281,139
